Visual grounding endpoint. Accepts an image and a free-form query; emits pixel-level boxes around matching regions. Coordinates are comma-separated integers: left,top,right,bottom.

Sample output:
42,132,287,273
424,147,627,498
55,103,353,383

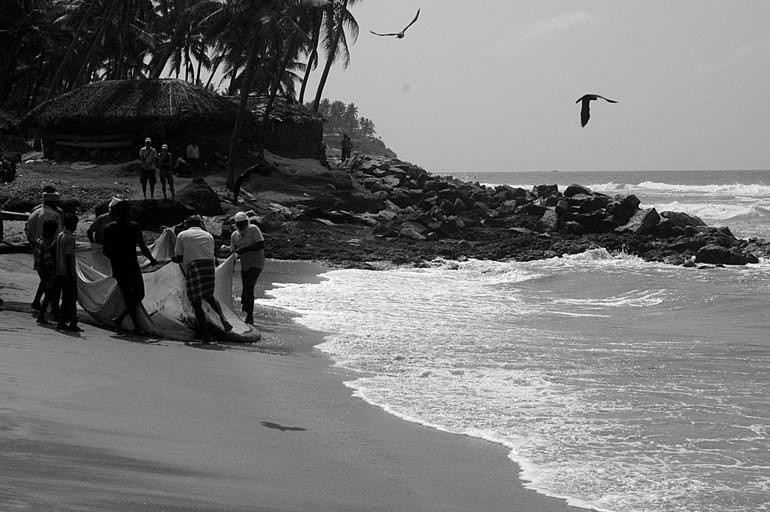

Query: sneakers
112,316,150,336
29,305,84,332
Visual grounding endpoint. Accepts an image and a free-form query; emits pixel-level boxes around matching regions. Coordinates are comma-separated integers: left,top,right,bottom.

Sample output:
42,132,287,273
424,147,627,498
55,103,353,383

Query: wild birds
576,93,619,129
366,8,422,42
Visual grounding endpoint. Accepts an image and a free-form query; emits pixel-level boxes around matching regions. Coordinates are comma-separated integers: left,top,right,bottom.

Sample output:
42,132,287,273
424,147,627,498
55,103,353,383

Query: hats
162,144,168,149
108,197,122,207
145,137,151,143
235,212,248,222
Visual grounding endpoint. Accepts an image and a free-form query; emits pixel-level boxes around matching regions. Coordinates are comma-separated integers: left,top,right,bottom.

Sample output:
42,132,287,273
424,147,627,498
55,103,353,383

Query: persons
170,216,234,343
24,193,64,313
340,134,350,163
345,137,353,158
157,143,178,202
98,198,159,338
50,212,86,332
29,185,64,222
229,210,266,325
186,140,202,180
86,194,124,245
213,149,222,167
138,137,158,199
217,152,229,167
172,152,189,178
30,219,70,332
